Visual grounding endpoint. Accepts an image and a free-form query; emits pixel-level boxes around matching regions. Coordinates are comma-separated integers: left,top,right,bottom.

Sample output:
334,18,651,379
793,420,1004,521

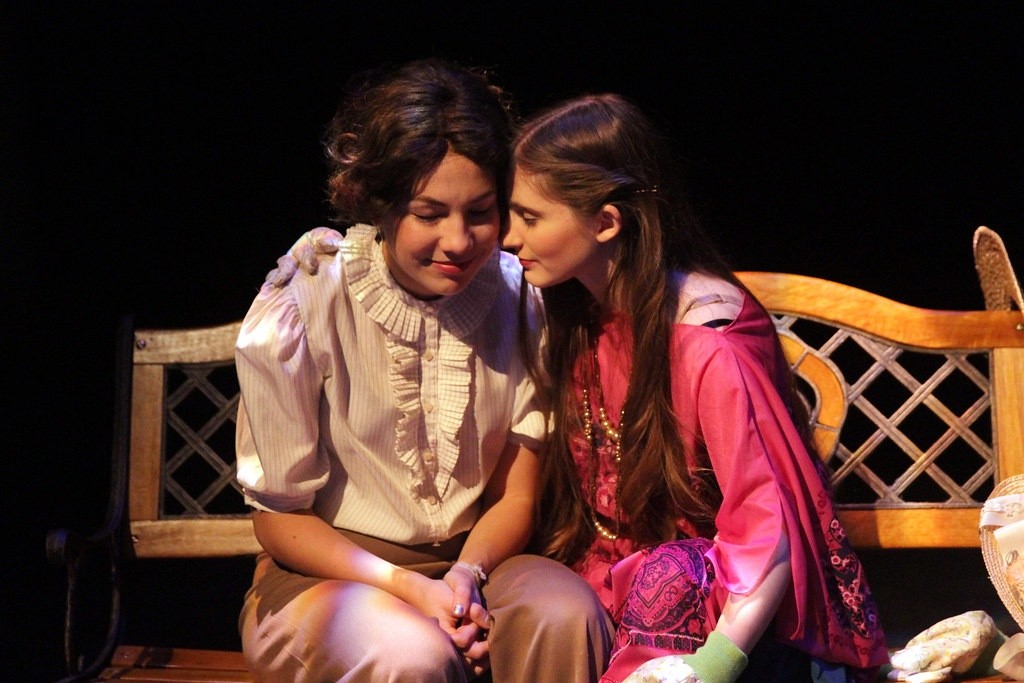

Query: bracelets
457,562,487,585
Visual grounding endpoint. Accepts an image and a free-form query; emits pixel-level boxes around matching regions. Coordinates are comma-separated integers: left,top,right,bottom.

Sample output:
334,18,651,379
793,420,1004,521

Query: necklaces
580,338,626,541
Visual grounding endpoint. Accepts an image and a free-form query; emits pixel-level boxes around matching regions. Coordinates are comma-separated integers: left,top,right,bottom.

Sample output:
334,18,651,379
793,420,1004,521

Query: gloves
620,631,748,683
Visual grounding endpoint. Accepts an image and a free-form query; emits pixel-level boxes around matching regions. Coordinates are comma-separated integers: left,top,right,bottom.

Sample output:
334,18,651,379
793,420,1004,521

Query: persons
271,94,890,683
233,63,615,683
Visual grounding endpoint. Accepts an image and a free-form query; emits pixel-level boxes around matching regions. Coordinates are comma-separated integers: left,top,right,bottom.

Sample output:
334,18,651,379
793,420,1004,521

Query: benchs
44,225,1024,683
